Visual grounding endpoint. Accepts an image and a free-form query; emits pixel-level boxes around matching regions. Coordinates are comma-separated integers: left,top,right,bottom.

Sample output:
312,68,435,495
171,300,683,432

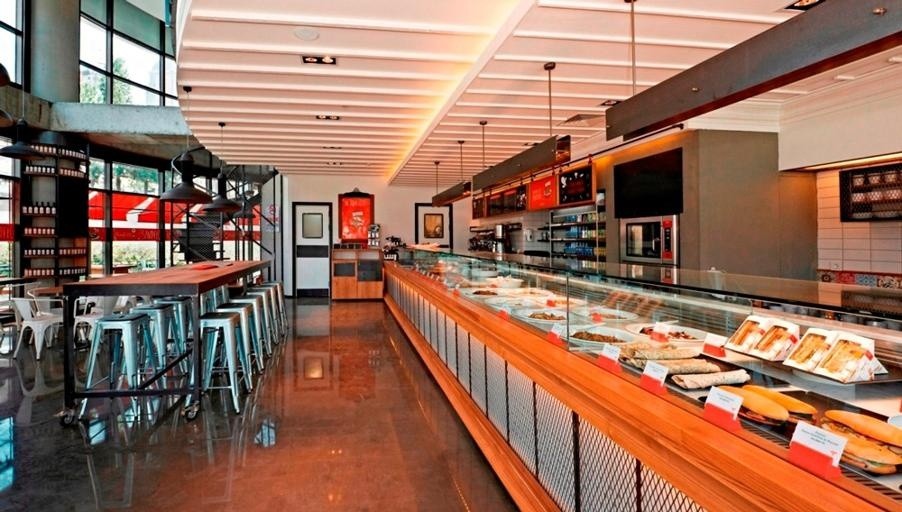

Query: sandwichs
730,319,864,372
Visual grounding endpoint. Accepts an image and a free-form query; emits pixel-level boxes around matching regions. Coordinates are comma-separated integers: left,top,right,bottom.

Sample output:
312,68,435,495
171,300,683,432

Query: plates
468,286,712,350
848,210,900,220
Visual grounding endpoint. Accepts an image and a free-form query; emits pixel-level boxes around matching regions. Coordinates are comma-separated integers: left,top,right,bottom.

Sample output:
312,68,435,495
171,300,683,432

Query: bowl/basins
850,191,902,202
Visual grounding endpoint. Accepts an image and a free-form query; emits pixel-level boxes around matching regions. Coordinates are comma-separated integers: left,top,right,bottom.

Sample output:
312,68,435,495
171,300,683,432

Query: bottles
22,200,56,215
550,212,606,257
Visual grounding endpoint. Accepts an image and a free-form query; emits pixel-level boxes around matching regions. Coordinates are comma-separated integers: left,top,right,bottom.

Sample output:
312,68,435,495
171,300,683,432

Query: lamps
160,86,256,219
0,1,49,164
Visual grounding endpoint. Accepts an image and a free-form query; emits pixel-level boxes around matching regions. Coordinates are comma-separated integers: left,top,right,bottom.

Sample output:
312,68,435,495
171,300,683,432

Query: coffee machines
382,236,401,261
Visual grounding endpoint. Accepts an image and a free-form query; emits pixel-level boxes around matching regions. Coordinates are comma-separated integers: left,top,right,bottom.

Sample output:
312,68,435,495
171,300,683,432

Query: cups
852,170,898,186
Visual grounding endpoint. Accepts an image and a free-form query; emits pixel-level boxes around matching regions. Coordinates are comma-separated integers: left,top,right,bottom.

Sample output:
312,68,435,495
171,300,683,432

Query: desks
60,257,274,427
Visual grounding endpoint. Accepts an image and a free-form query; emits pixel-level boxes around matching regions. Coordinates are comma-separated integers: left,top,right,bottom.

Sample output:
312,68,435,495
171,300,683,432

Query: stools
69,279,289,418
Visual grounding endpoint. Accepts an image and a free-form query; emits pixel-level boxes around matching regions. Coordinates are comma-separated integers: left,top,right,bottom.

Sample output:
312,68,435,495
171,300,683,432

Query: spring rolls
618,342,751,389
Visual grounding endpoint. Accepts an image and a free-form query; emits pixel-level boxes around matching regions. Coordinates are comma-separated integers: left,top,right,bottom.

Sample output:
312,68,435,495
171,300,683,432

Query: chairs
11,284,152,361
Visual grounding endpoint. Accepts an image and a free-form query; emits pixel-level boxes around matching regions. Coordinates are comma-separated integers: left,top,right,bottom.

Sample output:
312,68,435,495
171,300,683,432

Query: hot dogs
716,382,902,475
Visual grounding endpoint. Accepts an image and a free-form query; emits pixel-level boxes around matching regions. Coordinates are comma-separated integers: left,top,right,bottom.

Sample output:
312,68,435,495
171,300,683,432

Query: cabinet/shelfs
333,247,385,301
383,210,902,512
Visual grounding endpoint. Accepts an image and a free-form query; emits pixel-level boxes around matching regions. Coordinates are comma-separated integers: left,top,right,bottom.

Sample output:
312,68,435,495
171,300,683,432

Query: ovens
627,265,679,285
619,216,678,263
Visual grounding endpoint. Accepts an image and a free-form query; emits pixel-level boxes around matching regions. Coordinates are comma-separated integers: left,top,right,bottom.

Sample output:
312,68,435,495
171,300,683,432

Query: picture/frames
414,202,453,249
